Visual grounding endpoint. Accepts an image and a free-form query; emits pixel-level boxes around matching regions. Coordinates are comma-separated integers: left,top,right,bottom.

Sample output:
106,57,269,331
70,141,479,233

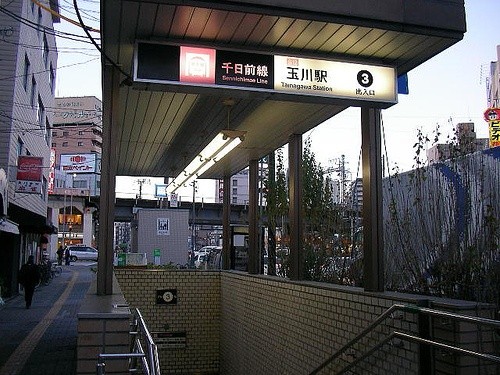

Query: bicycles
34,262,64,288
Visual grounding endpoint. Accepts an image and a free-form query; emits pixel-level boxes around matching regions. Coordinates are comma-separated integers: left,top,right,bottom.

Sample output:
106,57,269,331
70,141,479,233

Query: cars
196,246,217,263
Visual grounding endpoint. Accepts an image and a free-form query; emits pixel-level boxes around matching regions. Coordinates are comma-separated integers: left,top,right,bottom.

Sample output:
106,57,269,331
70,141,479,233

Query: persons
56,245,64,265
18,255,41,308
64,246,71,266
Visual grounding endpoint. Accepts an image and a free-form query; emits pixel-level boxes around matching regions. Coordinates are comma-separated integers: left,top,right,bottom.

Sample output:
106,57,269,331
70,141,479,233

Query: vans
67,245,99,261
